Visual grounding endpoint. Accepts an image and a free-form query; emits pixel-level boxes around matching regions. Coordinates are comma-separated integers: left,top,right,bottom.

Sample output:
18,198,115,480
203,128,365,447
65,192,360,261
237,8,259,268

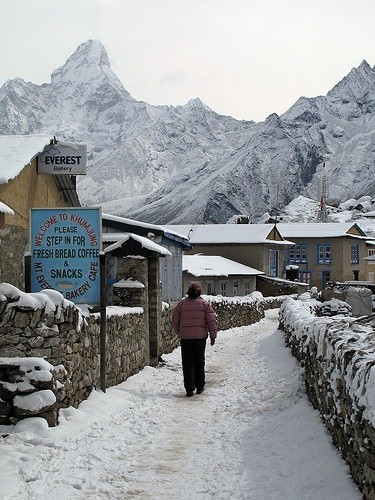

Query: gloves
211,338,215,345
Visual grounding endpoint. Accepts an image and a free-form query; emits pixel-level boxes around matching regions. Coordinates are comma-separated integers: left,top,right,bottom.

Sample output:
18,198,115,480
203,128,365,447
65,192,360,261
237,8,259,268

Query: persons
172,283,217,398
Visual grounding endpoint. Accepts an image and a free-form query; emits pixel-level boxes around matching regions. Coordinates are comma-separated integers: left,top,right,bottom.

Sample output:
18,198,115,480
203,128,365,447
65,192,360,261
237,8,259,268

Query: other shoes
196,388,204,394
186,393,194,397
